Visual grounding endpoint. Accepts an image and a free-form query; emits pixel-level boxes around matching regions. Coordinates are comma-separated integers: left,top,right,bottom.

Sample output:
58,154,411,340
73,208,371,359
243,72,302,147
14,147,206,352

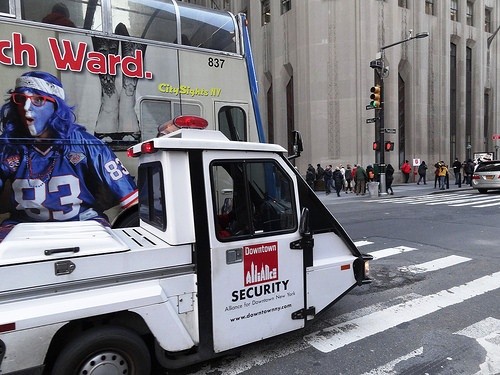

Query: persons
339,163,368,195
306,163,315,191
323,165,332,193
452,158,462,186
366,164,394,195
433,161,448,190
316,164,324,191
417,161,428,185
0,71,139,242
332,167,343,197
42,2,76,28
401,160,411,183
461,158,480,185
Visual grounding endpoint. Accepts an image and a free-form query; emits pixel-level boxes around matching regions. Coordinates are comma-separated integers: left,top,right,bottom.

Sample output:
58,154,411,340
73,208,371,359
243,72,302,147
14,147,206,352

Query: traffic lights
370,85,380,108
385,142,394,151
373,142,378,150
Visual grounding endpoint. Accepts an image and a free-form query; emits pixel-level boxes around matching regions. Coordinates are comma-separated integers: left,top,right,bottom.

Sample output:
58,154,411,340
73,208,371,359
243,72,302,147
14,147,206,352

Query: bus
0,0,303,244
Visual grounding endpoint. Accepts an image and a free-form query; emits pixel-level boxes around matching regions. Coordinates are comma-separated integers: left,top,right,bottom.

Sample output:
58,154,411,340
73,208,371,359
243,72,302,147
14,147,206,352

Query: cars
472,160,500,194
0,116,374,375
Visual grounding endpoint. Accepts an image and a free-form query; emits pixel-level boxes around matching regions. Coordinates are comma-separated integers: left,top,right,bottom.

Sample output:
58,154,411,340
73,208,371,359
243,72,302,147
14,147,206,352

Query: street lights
378,31,429,196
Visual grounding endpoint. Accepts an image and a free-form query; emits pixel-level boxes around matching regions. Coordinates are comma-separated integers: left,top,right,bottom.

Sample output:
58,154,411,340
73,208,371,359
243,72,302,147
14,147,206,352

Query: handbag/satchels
350,179,355,188
345,181,348,187
369,172,374,178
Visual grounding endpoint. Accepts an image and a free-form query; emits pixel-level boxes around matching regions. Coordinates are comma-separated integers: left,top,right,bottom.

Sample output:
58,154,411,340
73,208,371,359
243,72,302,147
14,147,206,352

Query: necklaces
20,149,55,186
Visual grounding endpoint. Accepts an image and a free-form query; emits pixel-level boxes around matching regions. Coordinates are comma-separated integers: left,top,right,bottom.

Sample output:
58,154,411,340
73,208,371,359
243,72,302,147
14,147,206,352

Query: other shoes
345,191,348,194
417,183,419,185
337,194,340,197
441,188,445,190
390,193,393,195
424,183,426,184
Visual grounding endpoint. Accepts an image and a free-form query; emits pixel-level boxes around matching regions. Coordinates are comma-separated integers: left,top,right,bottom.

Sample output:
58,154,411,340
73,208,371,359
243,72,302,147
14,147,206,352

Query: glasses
12,93,57,106
